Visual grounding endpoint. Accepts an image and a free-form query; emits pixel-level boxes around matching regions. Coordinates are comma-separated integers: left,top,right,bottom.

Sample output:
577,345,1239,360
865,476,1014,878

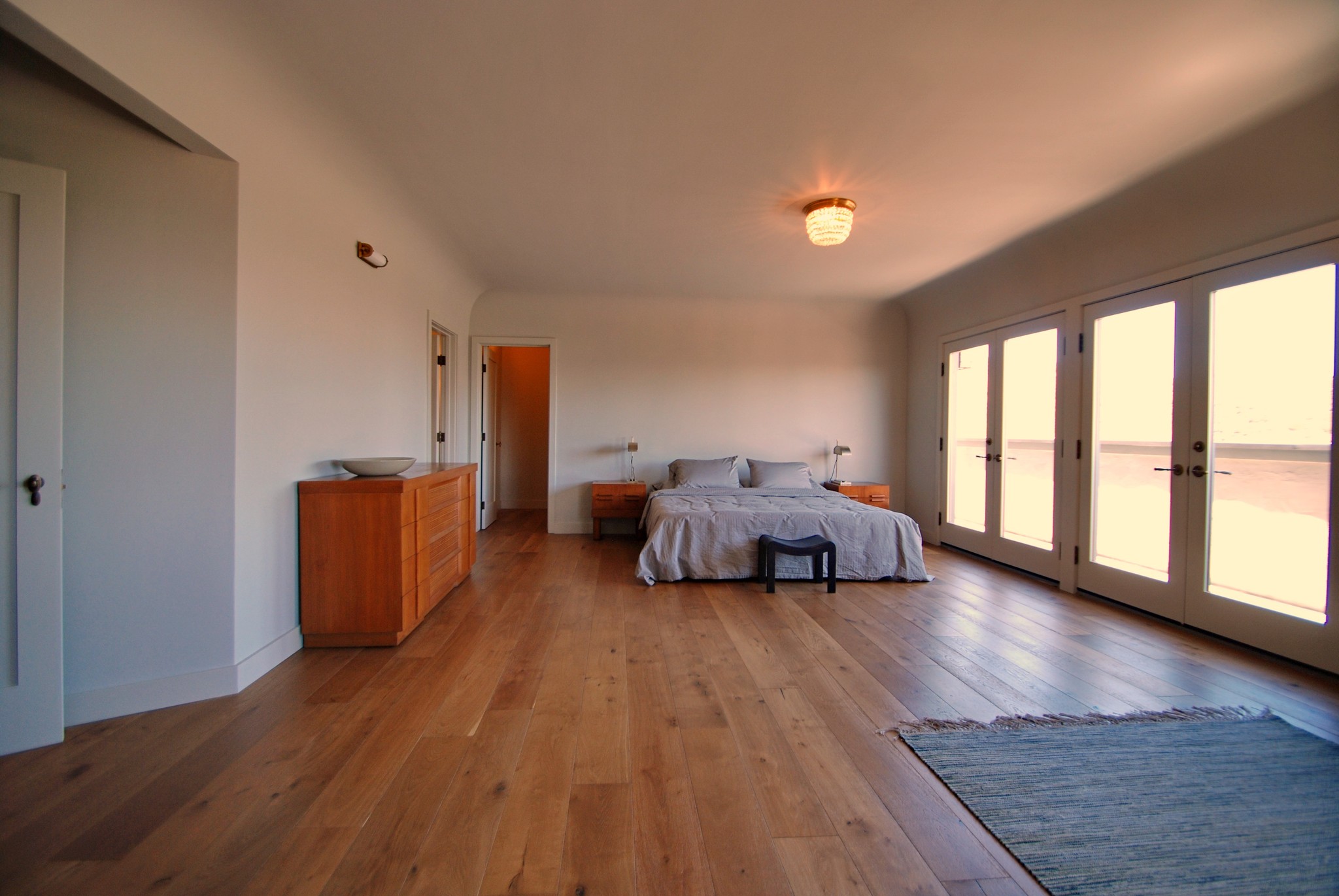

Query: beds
635,481,936,587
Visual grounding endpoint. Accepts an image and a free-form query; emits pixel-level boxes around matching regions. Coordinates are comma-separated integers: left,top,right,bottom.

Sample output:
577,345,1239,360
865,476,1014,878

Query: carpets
874,706,1339,896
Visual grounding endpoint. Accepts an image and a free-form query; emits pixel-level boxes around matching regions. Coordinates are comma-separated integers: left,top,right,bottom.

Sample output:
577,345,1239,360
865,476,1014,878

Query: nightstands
824,481,889,510
592,481,646,541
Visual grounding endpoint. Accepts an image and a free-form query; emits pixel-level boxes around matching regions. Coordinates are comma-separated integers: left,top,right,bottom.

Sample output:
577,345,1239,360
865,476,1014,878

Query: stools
758,534,836,594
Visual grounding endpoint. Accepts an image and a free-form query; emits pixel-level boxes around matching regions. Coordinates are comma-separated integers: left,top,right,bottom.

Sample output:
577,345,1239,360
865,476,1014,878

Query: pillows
667,456,740,488
746,459,813,489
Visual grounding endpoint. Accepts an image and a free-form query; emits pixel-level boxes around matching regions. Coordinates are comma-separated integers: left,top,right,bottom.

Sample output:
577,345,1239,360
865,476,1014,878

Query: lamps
628,436,638,483
357,241,389,268
802,198,856,246
831,440,852,485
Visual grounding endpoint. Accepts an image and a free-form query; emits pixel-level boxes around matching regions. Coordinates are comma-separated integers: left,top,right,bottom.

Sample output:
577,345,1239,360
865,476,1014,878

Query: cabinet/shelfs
298,462,478,647
